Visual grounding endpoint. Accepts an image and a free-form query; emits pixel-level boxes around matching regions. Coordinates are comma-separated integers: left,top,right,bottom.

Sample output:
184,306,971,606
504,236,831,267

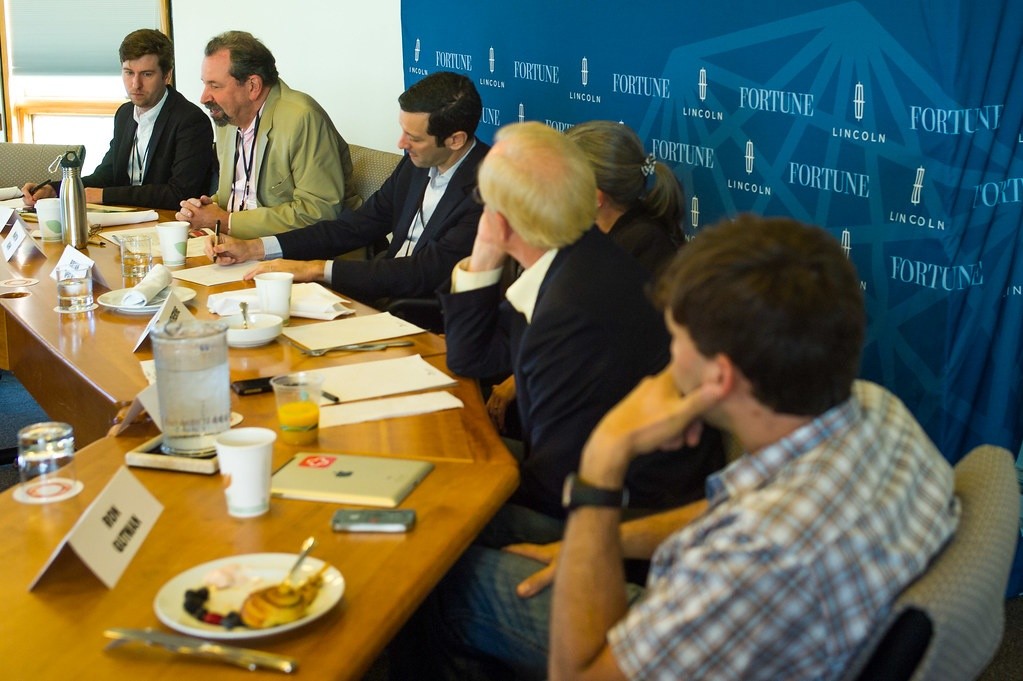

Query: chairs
0,141,86,195
347,144,404,203
841,444,1022,681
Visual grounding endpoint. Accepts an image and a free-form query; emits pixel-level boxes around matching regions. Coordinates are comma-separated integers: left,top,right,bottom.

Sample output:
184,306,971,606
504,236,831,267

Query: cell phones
232,376,273,395
331,508,416,533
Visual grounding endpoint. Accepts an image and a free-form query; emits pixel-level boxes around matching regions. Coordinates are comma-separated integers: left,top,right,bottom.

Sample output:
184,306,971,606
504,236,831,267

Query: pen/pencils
19,179,51,198
323,392,338,402
213,220,221,263
89,240,106,245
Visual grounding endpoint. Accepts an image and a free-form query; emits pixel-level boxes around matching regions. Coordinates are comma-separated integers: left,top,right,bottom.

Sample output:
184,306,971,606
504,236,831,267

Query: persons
175,31,366,261
203,71,492,306
436,119,727,546
386,215,963,681
485,120,685,430
22,28,220,213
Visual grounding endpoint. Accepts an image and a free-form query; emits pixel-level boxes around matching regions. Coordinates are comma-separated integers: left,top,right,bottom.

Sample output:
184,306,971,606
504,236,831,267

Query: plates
97,285,196,313
220,312,283,348
152,551,346,640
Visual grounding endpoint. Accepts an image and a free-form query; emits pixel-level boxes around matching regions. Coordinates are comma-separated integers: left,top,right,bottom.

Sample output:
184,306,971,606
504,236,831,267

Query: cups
270,369,325,445
157,220,191,266
120,234,153,279
33,198,63,243
15,422,74,497
253,272,294,328
215,427,276,519
148,319,232,457
55,263,95,311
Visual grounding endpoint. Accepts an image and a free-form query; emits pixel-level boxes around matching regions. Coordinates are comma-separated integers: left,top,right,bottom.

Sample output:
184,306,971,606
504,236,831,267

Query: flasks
48,151,88,251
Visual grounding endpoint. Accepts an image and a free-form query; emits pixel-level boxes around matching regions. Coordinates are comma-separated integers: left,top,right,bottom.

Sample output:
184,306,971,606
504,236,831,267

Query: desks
0,201,521,680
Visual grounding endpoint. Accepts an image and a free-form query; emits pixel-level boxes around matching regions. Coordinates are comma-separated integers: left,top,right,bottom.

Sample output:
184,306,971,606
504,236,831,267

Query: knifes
100,628,296,674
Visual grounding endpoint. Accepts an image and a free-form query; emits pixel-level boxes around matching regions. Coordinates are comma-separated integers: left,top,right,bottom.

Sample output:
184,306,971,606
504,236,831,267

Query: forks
290,342,389,356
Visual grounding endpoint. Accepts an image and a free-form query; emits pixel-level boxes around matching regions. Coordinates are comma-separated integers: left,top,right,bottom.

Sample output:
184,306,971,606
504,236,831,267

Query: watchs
562,473,629,509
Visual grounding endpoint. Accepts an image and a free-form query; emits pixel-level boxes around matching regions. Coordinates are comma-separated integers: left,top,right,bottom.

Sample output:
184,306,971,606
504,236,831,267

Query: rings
494,405,497,409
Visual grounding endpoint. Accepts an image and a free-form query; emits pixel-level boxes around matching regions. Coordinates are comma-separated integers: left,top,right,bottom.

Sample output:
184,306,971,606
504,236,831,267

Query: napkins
87,209,159,228
122,263,173,307
206,282,357,320
